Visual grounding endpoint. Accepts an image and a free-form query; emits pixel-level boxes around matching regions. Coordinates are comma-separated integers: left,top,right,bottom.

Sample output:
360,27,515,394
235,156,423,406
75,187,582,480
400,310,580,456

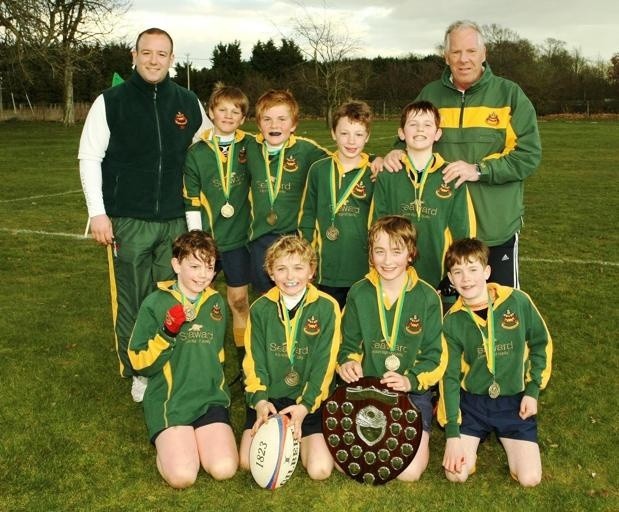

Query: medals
220,202,234,220
184,307,196,322
266,210,278,225
284,371,300,387
489,382,500,399
385,354,401,371
414,202,423,217
325,226,339,241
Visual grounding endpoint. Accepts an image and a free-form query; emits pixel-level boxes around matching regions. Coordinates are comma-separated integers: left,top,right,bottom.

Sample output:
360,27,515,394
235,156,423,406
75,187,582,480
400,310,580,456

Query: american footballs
249,412,300,489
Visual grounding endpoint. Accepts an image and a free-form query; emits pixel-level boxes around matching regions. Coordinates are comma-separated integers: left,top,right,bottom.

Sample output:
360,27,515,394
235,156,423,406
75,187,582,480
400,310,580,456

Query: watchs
477,164,481,176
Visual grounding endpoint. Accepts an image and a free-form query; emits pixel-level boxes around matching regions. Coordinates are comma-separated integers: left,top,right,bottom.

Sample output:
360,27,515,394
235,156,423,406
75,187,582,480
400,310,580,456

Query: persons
240,235,334,480
126,227,242,488
368,101,476,291
77,29,214,403
245,87,384,296
439,238,553,487
297,98,382,311
184,82,257,375
382,20,543,290
334,216,448,483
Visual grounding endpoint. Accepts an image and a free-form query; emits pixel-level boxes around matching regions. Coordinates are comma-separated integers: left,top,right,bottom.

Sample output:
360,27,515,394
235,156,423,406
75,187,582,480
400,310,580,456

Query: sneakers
129,375,150,403
